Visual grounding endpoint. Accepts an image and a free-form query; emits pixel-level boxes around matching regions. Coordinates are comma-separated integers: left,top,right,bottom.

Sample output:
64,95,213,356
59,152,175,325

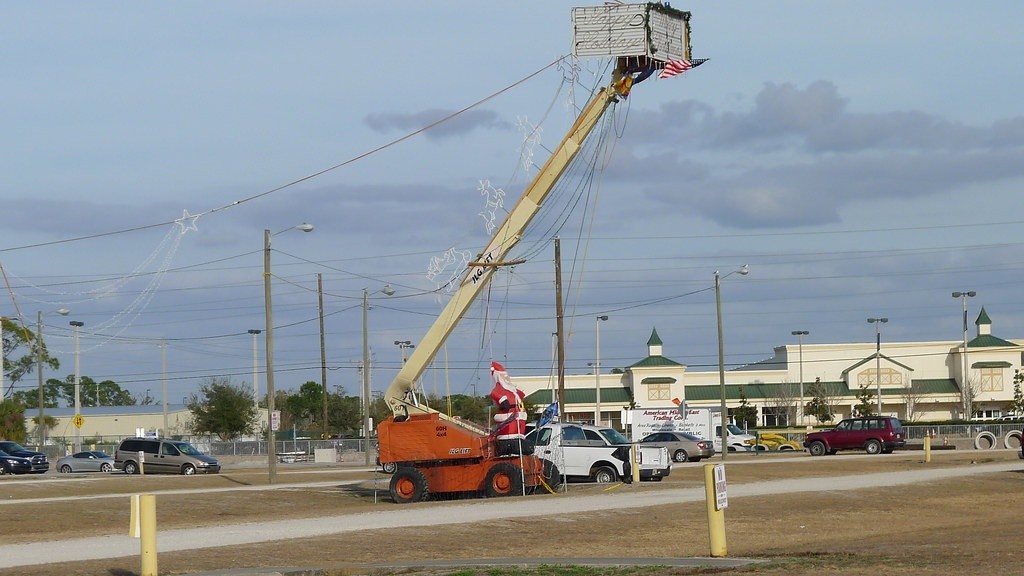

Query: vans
114,437,220,476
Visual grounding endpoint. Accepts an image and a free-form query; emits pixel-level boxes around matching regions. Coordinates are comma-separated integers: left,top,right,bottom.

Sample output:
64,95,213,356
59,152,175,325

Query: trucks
632,406,756,453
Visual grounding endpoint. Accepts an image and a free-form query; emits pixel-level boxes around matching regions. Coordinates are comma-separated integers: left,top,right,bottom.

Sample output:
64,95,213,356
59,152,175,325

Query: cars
56,450,121,473
634,432,715,462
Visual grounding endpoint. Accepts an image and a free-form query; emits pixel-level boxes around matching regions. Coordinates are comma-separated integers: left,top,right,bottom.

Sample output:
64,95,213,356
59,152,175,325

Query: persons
491,362,535,455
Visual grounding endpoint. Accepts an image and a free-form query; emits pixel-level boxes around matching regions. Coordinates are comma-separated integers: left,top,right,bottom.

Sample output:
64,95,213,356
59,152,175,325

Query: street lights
867,318,888,416
587,362,601,375
249,329,261,432
364,288,396,466
597,315,608,426
792,330,809,426
952,291,976,438
38,308,70,445
394,340,414,369
715,270,748,460
70,321,85,454
264,224,314,484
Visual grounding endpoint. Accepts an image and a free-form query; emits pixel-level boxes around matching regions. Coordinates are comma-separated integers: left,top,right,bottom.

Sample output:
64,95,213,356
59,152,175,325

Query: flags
537,402,558,429
659,58,710,78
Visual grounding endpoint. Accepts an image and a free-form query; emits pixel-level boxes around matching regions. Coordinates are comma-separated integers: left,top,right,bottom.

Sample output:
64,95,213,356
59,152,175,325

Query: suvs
524,422,673,483
0,441,49,475
803,415,906,456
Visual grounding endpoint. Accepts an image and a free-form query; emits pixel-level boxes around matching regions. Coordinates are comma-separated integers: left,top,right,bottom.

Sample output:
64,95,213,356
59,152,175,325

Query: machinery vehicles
376,2,712,504
